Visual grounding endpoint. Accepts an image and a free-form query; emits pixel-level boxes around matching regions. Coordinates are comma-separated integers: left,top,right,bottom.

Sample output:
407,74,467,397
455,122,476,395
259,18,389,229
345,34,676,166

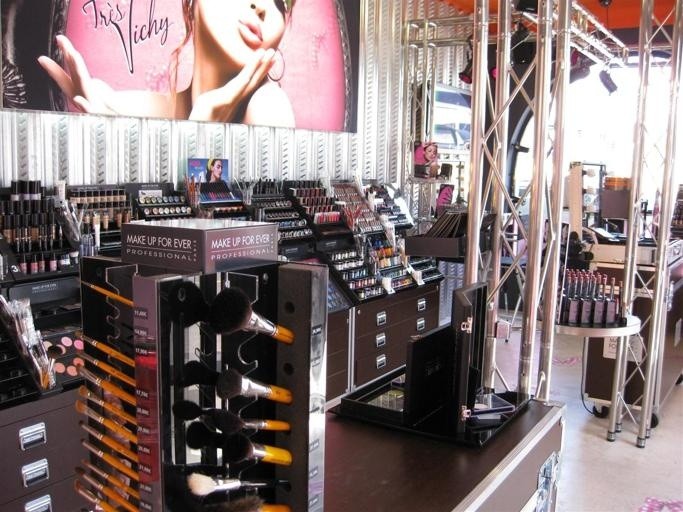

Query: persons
423,143,438,166
205,159,225,183
36,0,298,129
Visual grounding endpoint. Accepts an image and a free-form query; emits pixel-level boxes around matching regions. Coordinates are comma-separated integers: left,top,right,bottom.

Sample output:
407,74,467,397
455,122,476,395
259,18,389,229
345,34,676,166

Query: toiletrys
558,269,624,324
181,178,445,302
0,177,190,275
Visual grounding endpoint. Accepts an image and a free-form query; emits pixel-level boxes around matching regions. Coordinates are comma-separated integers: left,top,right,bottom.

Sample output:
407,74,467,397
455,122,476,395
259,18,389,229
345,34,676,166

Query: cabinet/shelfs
0,173,493,418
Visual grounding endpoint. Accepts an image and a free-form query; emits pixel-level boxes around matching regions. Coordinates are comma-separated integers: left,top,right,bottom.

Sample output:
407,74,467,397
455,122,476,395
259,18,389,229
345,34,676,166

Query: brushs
0,293,58,395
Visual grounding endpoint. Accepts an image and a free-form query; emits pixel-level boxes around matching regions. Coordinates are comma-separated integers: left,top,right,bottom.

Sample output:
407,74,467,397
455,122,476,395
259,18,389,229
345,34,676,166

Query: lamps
460,30,474,85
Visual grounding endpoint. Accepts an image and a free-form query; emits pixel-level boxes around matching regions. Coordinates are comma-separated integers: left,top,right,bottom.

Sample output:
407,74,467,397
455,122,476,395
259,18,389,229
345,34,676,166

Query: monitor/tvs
425,80,472,151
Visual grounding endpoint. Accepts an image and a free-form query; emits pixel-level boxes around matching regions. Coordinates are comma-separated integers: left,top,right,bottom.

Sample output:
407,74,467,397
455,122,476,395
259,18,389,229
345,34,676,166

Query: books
424,208,497,238
